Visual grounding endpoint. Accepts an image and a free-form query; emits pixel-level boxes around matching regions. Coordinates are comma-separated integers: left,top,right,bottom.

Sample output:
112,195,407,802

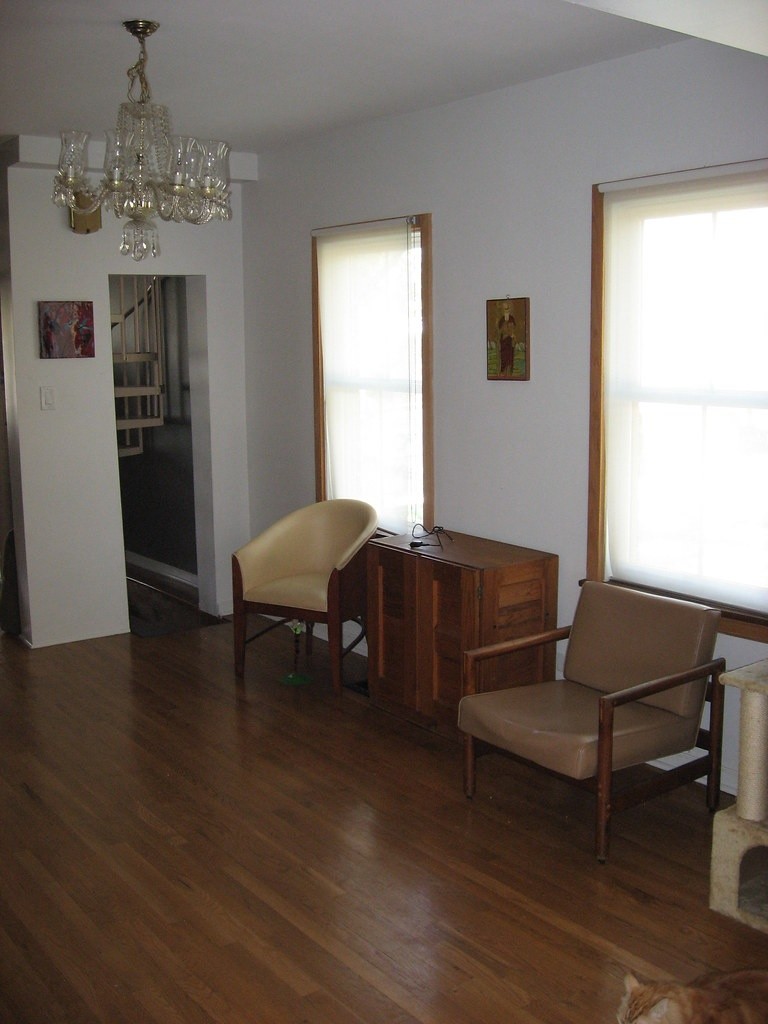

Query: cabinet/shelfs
365,530,559,759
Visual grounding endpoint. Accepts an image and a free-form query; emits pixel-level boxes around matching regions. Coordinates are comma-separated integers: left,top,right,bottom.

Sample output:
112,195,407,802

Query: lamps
53,20,231,261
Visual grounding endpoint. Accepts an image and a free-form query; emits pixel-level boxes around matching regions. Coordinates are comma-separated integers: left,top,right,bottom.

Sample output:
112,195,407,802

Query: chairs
232,499,378,696
457,582,726,865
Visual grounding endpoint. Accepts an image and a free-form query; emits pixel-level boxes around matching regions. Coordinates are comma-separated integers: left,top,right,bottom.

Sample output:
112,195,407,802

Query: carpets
126,578,229,640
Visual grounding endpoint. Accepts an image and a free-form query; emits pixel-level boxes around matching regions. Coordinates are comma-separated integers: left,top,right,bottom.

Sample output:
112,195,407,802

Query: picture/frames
486,297,531,381
